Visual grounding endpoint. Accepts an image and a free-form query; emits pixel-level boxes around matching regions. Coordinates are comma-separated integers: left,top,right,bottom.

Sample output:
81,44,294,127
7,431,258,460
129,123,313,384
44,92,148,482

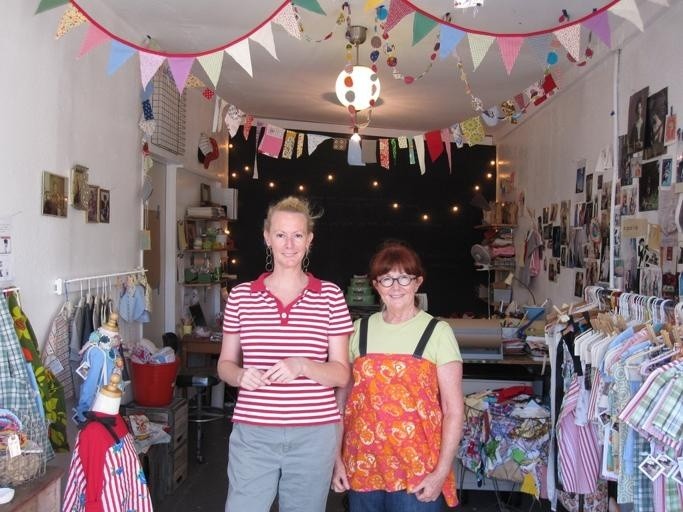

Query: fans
471,244,491,265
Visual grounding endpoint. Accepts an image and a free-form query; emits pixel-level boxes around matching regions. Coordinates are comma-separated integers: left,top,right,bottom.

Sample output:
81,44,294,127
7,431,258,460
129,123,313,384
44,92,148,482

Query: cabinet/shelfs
126,399,189,495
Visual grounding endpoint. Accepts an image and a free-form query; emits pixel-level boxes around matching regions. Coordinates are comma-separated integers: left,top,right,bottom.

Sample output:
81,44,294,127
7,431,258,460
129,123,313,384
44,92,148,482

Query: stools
175,371,227,462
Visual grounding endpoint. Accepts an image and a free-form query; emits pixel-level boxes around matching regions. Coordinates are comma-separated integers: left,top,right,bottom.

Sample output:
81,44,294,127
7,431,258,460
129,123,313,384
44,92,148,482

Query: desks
181,333,222,369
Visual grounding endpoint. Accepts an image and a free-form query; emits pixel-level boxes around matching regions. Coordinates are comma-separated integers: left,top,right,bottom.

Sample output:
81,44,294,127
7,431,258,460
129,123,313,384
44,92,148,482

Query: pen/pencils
499,310,529,328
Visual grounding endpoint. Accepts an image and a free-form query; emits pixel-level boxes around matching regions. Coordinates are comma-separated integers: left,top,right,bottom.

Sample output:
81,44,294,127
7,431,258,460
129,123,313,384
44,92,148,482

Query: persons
215,195,354,512
635,238,645,267
630,98,642,147
329,241,463,512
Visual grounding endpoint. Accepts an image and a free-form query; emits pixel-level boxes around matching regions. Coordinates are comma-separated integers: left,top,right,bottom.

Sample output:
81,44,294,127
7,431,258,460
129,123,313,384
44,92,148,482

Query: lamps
335,25,380,110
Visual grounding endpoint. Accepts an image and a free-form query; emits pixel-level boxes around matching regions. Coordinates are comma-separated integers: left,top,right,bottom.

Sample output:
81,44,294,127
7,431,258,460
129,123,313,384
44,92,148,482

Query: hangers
545,284,683,389
49,275,113,329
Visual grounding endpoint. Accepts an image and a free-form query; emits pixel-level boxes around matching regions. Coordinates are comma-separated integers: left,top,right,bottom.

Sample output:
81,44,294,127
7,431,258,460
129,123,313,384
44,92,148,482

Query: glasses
375,274,418,288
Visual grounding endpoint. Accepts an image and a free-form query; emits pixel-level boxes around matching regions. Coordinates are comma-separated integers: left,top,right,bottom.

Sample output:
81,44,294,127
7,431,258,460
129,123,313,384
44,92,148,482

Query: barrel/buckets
129,356,180,407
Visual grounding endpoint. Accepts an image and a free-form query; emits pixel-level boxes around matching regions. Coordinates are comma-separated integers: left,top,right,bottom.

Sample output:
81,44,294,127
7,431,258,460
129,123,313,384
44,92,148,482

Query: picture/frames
41,164,110,224
